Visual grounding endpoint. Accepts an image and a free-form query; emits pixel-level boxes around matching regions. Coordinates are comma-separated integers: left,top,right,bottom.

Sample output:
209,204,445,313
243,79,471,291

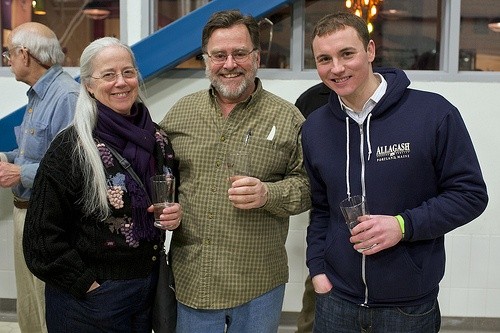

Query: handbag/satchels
147,264,178,333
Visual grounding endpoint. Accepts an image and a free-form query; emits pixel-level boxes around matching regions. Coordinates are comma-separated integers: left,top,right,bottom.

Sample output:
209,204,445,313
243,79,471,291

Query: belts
12,195,30,209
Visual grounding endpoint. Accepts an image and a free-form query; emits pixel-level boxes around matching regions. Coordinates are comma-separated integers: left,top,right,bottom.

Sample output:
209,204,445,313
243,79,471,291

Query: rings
177,218,180,223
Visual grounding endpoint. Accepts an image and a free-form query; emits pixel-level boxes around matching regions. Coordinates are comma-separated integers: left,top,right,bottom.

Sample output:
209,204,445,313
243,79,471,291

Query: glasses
89,67,138,82
205,46,259,65
2,45,31,61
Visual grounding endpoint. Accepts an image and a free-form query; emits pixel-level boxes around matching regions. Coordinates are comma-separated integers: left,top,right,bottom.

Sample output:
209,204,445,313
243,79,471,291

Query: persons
300,13,489,333
294,82,332,333
157,10,312,333
0,22,82,333
21,37,182,333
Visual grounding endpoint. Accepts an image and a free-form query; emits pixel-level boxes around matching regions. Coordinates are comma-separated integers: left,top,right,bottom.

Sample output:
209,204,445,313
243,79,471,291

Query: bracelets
395,215,405,240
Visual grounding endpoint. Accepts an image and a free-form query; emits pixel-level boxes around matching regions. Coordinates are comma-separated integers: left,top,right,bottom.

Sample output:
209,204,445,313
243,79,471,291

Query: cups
225,150,253,189
149,174,175,229
338,195,379,253
